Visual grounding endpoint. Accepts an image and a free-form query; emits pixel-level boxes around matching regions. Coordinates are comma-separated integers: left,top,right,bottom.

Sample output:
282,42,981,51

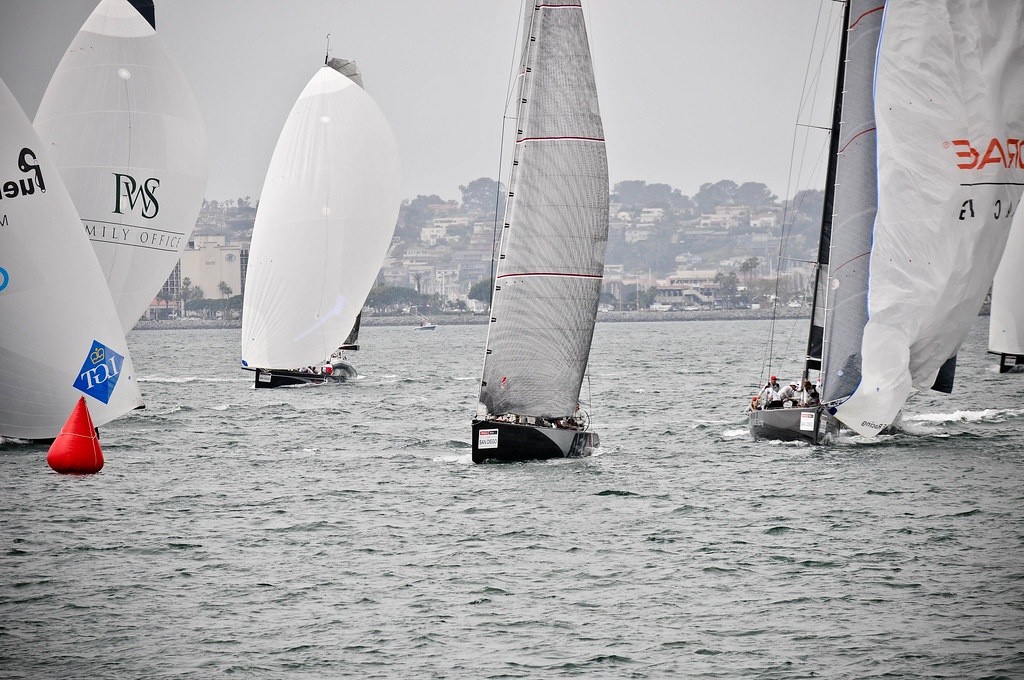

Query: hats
790,382,798,386
771,375,777,380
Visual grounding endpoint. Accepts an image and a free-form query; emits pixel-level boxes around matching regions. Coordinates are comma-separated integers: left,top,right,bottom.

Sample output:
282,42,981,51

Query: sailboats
0,1,208,443
471,0,610,465
748,1,1023,444
241,64,405,390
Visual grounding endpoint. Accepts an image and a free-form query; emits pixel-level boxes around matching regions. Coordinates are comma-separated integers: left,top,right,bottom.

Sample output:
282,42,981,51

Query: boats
413,324,434,330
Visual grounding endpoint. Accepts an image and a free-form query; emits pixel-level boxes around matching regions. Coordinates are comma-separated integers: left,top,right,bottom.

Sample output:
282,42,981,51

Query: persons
299,352,346,375
568,403,587,430
746,376,820,415
